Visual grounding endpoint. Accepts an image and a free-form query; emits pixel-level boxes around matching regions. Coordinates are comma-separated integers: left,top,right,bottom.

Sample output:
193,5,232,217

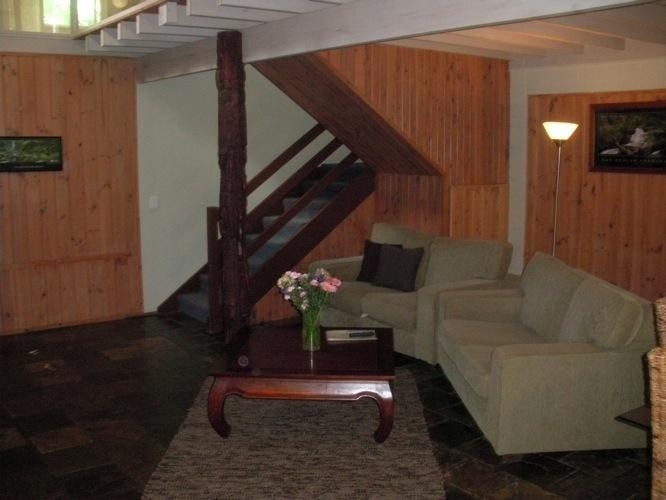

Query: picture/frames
588,100,666,175
0,136,62,172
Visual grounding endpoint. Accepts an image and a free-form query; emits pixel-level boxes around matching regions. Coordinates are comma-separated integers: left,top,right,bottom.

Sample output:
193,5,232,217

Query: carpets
140,368,447,500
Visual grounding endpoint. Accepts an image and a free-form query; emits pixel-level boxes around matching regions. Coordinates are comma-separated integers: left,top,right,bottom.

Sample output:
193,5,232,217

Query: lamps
542,121,579,257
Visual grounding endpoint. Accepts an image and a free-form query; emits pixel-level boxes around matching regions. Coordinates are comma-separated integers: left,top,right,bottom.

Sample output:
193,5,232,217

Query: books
326,329,377,341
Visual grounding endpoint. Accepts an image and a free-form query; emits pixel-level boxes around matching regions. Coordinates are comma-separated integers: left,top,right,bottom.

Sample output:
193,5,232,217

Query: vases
303,312,320,352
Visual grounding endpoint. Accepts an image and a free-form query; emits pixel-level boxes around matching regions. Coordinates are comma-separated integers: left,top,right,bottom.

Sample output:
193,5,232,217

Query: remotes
350,330,376,337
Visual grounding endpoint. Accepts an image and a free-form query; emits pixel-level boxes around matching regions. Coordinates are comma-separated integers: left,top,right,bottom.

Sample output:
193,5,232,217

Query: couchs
436,250,658,465
309,222,514,367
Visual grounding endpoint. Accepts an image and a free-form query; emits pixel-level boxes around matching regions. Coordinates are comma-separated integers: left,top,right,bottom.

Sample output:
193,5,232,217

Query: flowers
277,268,342,344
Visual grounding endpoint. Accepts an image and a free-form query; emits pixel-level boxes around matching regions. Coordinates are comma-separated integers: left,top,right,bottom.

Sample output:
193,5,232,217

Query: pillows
371,242,424,293
356,240,403,282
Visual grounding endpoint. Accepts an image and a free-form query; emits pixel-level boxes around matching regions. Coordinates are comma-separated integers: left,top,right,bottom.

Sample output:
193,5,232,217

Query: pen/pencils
350,332,375,336
